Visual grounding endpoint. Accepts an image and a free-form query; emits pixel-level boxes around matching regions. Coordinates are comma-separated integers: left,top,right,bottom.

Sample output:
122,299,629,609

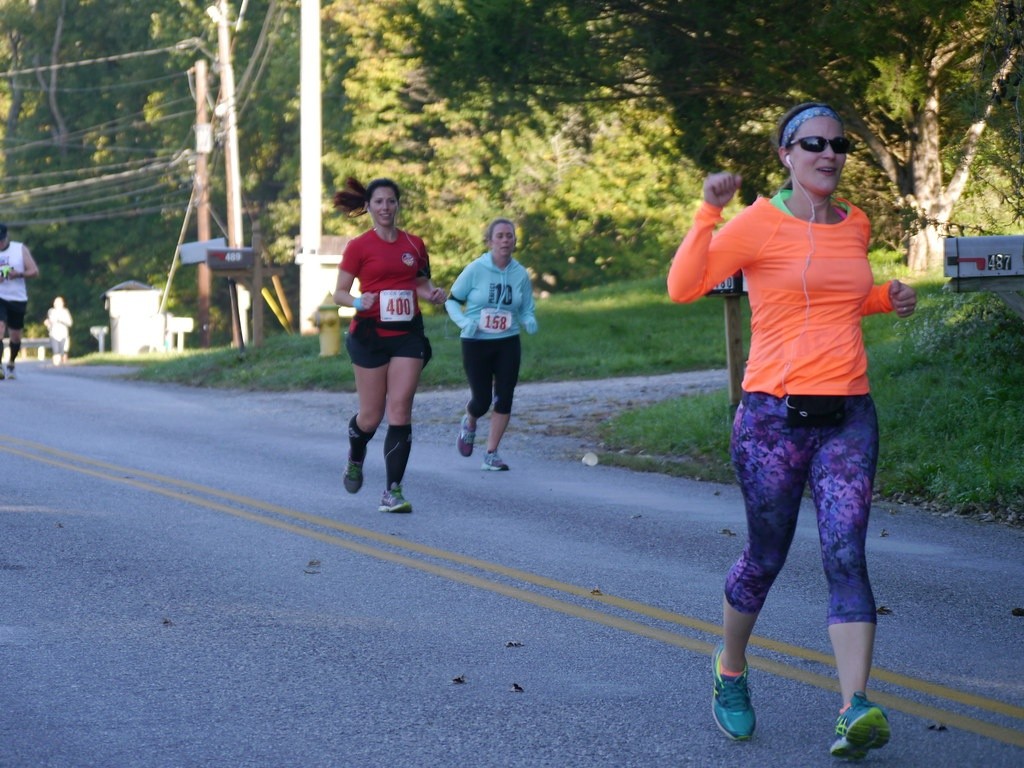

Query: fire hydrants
306,290,341,357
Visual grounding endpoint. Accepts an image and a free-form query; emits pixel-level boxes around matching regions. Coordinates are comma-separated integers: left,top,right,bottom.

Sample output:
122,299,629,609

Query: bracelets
353,298,365,311
20,272,23,278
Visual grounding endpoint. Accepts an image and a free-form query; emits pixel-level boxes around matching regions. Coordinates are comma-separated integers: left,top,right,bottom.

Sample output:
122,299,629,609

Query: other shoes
5,365,14,379
0,366,4,379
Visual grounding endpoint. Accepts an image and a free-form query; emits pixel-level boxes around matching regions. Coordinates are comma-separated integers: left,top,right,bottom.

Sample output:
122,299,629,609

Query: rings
903,307,907,311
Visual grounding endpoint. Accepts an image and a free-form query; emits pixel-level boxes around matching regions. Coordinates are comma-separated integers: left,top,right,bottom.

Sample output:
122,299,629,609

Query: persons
444,220,537,473
45,297,75,364
666,101,918,757
331,178,443,515
0,222,38,380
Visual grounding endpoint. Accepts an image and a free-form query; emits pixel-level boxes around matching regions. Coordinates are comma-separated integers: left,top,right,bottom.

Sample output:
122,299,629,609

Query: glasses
785,136,851,154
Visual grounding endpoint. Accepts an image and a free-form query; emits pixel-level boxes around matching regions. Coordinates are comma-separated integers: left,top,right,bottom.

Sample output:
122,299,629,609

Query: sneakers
457,414,476,457
342,448,367,492
378,483,412,514
481,450,509,471
830,692,892,762
710,645,756,739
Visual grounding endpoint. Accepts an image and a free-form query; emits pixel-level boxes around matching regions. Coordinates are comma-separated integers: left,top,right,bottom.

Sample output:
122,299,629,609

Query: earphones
786,154,795,171
367,206,370,213
